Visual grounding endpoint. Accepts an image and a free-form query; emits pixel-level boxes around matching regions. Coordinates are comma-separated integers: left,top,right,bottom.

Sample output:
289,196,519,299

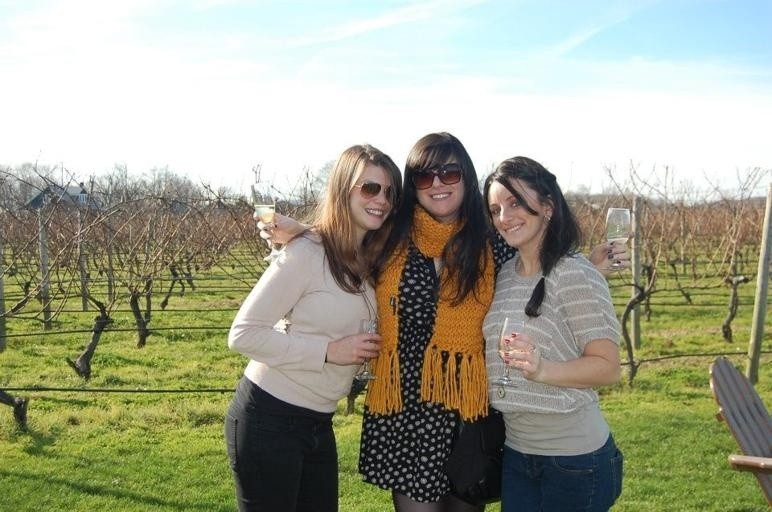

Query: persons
479,155,625,512
224,143,403,512
251,129,632,512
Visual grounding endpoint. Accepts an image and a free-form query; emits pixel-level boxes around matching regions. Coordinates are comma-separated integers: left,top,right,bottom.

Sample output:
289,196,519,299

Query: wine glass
491,314,527,386
602,206,632,272
354,318,379,381
250,180,282,263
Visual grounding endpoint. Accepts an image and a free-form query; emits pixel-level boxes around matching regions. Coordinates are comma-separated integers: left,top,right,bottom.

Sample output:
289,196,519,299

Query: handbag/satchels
448,404,507,505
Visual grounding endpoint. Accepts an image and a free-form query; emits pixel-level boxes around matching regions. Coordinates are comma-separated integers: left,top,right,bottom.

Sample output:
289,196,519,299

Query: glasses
411,163,461,190
354,181,392,204
